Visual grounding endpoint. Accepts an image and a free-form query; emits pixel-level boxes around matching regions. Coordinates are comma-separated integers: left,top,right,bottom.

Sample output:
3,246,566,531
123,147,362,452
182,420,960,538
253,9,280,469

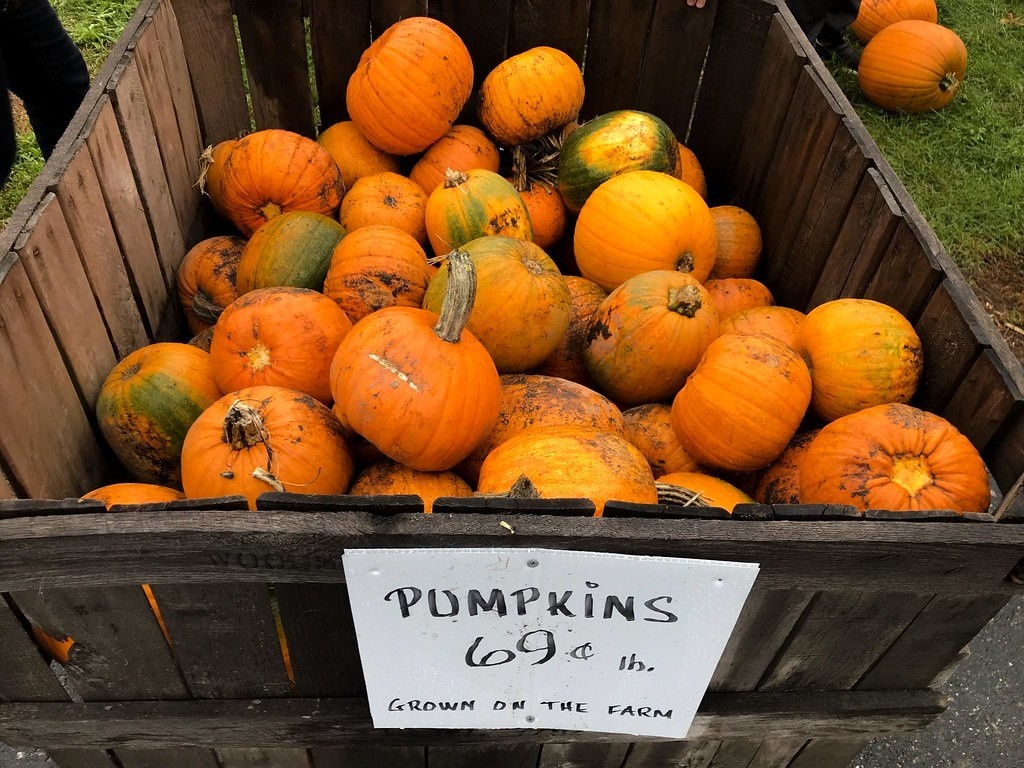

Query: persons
1,1,90,188
684,0,862,69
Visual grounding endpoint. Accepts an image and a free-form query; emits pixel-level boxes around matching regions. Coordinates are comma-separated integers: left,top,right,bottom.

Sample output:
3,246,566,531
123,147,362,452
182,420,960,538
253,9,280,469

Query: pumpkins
34,0,990,683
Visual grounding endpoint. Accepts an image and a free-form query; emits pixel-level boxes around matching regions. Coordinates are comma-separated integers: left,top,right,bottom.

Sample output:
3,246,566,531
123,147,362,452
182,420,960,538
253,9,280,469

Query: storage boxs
0,0,1024,768
